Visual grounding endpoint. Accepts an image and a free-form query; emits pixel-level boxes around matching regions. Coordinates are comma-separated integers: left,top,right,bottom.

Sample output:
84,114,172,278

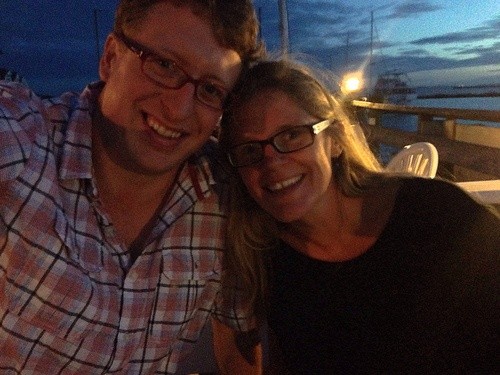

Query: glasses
227,120,329,168
115,32,235,111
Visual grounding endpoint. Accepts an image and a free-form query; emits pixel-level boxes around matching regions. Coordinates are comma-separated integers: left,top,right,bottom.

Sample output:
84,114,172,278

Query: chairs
382,142,439,179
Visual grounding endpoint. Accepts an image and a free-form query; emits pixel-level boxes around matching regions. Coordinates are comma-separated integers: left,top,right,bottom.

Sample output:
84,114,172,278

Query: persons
1,0,262,375
215,47,500,375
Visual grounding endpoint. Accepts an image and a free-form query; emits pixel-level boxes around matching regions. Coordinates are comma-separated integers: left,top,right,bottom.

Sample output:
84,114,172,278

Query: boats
369,71,417,105
